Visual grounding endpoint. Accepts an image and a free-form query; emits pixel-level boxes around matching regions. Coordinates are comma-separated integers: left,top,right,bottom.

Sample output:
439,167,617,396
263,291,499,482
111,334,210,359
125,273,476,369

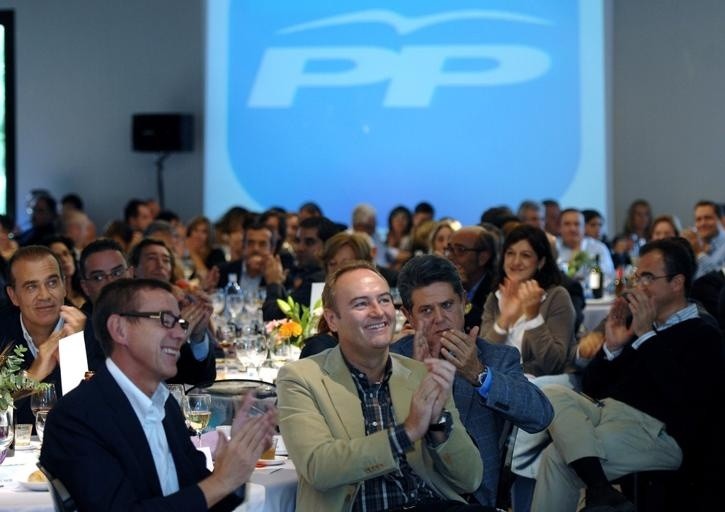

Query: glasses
630,271,675,287
83,264,129,283
106,308,190,335
442,243,482,260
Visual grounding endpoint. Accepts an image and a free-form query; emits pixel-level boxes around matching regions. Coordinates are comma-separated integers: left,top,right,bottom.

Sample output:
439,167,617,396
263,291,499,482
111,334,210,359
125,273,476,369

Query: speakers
132,114,195,151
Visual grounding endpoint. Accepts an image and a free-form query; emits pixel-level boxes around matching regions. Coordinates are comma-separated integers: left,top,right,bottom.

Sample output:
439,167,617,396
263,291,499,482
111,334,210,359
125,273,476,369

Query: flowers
566,248,603,277
262,296,325,353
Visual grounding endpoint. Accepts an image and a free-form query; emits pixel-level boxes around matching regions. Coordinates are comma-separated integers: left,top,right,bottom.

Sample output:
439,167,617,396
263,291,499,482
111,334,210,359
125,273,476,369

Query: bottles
588,254,605,298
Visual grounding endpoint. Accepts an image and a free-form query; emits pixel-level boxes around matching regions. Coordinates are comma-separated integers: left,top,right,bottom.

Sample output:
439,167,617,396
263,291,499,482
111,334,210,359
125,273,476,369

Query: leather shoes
580,486,636,512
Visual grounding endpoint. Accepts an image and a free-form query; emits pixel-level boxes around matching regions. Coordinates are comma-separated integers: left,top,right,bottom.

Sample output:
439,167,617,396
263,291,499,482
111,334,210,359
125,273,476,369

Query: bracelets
430,411,453,431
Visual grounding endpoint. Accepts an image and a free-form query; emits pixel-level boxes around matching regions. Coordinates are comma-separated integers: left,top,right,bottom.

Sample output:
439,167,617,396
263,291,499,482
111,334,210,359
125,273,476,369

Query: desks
582,293,618,329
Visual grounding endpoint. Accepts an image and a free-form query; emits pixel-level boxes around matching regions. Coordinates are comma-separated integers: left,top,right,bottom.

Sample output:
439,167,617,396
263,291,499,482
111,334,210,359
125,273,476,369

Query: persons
529,237,725,512
277,261,509,512
0,188,724,436
34,277,278,512
389,254,554,507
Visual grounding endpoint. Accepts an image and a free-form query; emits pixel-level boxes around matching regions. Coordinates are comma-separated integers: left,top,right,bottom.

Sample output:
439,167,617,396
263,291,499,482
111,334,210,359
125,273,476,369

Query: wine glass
207,291,292,382
166,381,214,452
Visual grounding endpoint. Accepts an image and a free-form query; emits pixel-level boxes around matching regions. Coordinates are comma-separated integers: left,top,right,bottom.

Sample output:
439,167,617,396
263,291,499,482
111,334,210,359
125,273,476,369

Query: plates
19,478,49,493
256,457,292,467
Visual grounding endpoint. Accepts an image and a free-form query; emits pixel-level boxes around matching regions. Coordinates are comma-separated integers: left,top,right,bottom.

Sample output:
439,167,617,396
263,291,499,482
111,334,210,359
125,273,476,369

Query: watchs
472,365,488,388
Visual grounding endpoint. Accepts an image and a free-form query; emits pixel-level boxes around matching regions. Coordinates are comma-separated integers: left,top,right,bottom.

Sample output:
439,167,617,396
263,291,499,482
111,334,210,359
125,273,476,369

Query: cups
15,423,35,450
30,380,56,443
263,439,278,458
